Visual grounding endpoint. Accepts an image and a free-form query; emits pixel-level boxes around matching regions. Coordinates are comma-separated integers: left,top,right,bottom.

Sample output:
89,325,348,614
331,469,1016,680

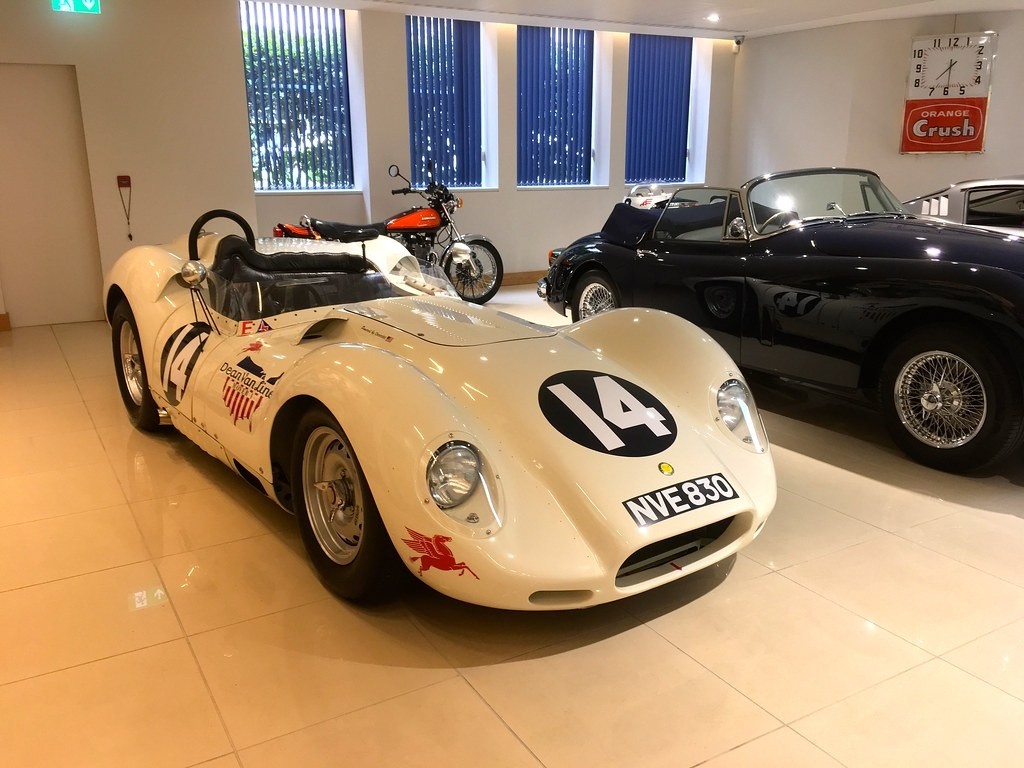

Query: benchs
206,235,397,321
600,197,799,240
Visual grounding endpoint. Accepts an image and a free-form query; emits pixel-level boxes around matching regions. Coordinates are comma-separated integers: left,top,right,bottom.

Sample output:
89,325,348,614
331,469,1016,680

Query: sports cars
102,209,778,611
535,165,1024,477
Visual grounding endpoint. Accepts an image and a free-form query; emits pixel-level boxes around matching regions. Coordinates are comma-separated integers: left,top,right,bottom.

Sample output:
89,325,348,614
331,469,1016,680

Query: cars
895,177,1024,240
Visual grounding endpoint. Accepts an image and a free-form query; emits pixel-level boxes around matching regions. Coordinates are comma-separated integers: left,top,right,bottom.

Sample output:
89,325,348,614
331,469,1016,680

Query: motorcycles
273,160,504,306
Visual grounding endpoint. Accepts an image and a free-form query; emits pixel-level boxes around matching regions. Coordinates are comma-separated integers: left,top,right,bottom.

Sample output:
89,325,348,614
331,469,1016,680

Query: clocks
898,31,999,154
